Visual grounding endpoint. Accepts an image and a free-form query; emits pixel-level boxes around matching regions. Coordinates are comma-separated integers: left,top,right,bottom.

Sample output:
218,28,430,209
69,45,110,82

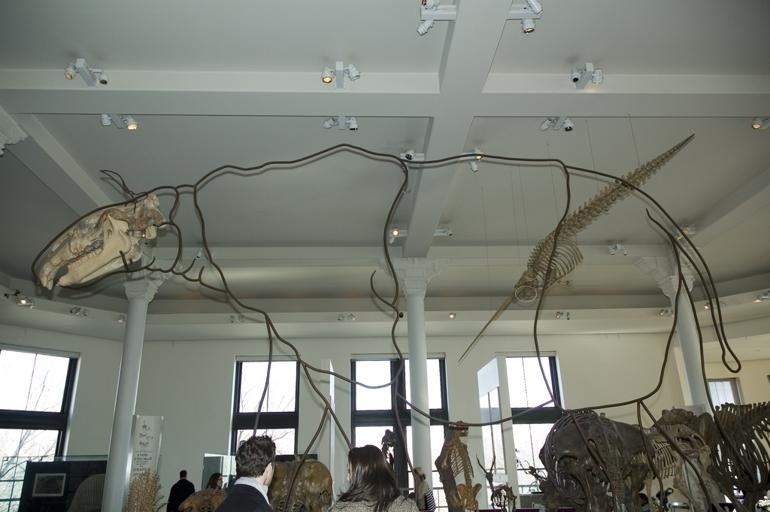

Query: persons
164,469,198,512
639,492,650,512
325,444,421,511
411,466,437,512
205,472,224,490
655,490,672,511
212,434,279,511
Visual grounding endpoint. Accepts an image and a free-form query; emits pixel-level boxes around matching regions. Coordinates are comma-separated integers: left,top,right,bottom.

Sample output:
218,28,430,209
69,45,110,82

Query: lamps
750,116,768,133
65,58,110,87
102,113,138,131
322,1,629,256
